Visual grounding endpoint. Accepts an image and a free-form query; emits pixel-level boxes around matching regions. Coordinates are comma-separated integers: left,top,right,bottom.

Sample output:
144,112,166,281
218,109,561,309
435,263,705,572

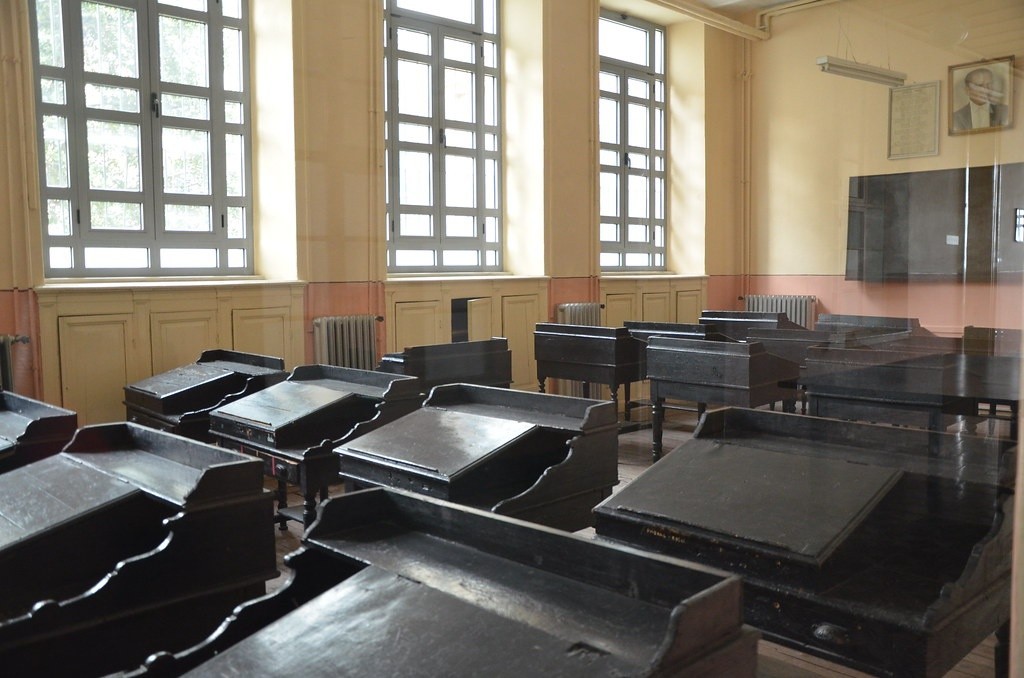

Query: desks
0,421,270,667
375,337,514,397
623,322,748,425
533,323,648,421
646,337,800,462
107,485,765,678
699,310,1021,442
208,364,419,531
592,406,1018,678
123,349,291,444
0,390,78,474
331,383,620,532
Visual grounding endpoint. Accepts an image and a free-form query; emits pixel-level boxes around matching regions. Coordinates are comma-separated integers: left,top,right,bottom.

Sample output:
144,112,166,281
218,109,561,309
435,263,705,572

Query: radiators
312,314,385,374
736,295,815,334
555,303,606,399
0,335,32,394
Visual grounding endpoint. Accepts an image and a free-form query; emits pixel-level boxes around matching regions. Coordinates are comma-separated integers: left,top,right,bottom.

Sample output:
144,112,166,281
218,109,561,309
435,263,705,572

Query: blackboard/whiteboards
843,162,1024,281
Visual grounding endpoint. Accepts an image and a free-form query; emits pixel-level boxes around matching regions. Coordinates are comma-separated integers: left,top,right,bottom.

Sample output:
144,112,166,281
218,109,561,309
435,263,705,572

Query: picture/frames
886,80,941,160
946,54,1018,137
